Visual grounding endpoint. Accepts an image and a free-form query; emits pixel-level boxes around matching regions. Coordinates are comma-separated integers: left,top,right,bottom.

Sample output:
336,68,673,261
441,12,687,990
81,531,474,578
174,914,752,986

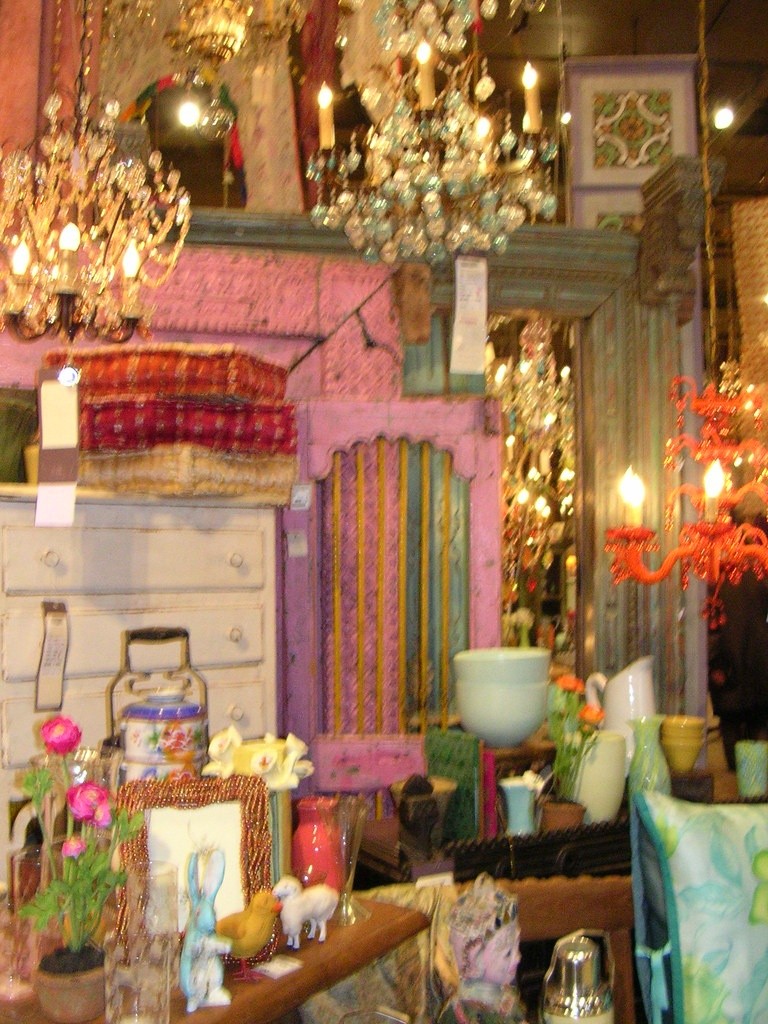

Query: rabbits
179,850,232,1012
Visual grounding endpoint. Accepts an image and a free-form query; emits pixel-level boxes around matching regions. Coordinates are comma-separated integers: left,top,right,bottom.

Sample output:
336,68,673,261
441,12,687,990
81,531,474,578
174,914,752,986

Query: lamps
298,0,563,269
0,0,194,389
484,313,577,596
603,0,767,629
103,1,312,140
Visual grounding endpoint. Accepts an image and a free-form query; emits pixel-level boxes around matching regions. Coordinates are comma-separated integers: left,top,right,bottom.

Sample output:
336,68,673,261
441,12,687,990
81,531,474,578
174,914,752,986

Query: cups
103,931,169,1024
289,867,327,888
30,746,124,852
125,860,181,993
733,742,768,797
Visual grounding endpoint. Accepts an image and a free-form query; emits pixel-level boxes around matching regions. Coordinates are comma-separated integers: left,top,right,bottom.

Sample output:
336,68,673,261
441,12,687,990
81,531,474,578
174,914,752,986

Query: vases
1,745,179,1024
292,799,344,890
454,645,705,838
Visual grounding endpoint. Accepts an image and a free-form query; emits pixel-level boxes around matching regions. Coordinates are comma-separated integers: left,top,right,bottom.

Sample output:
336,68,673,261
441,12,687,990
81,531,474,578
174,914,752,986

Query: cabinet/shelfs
0,482,291,899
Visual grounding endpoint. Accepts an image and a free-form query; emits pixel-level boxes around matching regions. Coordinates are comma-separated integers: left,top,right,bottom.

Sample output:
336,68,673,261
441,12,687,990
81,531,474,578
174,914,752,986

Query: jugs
541,929,616,1024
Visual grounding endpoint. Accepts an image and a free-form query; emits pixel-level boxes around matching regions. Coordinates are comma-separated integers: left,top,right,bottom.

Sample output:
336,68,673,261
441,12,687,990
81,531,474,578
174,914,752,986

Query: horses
272,875,339,948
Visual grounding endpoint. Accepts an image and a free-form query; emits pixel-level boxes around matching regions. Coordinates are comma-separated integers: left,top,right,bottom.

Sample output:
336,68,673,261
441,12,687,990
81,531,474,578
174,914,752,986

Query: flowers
12,713,145,955
546,673,604,802
200,725,315,790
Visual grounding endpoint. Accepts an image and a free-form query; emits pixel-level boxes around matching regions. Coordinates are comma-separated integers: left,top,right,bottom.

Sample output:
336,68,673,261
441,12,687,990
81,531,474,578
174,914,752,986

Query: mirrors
485,309,580,684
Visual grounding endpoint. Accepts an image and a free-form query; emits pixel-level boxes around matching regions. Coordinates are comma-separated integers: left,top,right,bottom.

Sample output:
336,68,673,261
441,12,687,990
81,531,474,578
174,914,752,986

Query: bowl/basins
455,684,549,751
451,645,553,684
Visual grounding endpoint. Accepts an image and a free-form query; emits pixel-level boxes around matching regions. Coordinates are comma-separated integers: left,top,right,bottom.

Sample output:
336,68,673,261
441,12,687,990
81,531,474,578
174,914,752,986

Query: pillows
636,788,767,1024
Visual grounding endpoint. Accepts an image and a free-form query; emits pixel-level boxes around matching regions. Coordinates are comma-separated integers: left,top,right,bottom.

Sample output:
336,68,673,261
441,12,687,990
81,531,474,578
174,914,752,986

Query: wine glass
316,797,371,927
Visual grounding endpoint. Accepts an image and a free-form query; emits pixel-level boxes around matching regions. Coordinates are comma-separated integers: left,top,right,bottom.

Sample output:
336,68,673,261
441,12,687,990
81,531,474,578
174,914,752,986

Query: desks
1,898,430,1024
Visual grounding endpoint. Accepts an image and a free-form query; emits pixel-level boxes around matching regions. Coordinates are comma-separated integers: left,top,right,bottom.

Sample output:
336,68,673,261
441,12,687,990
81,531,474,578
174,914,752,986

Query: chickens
216,892,283,982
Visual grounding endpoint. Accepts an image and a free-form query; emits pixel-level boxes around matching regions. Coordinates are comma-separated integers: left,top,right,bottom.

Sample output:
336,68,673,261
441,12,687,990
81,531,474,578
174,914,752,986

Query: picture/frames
564,55,698,190
115,774,276,970
572,189,710,721
